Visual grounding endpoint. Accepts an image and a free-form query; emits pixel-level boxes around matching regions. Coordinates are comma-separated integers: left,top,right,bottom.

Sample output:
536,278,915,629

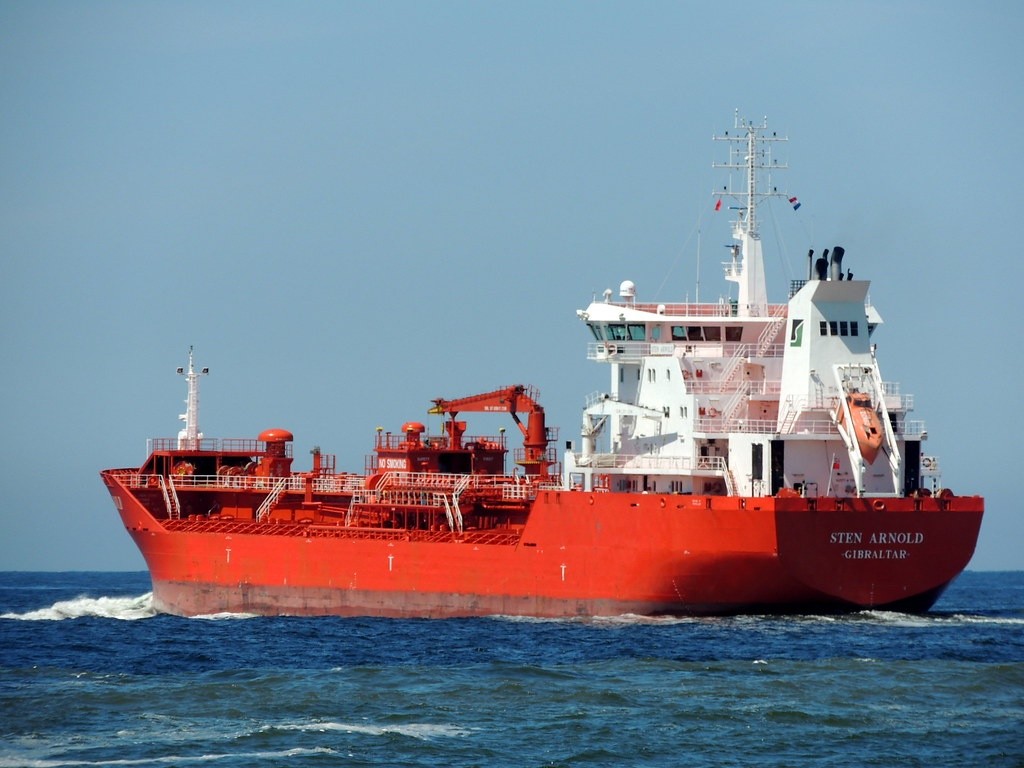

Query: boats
100,109,985,621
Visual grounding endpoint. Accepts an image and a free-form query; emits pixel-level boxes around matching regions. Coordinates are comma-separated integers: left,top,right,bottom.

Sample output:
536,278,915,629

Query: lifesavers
923,458,931,467
149,476,159,487
682,370,690,380
873,500,886,512
708,407,718,417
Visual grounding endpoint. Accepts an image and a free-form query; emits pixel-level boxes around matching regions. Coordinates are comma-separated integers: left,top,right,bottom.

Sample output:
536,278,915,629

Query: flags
715,200,721,211
789,197,801,210
